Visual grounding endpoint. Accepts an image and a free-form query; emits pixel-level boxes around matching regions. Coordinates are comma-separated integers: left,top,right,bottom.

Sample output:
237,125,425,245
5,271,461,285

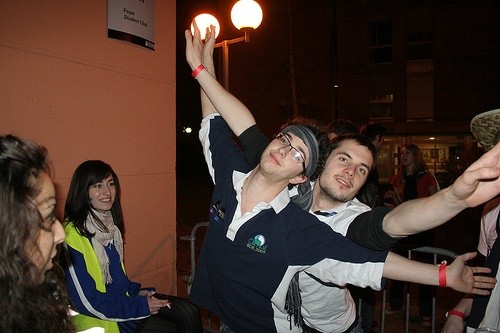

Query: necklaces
407,168,413,176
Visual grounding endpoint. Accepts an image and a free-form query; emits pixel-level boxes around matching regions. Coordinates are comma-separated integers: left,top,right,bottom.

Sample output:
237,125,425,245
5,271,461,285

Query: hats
470,109,500,151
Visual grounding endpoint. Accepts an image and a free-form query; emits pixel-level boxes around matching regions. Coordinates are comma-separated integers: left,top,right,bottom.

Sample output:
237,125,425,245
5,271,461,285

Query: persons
0,134,75,333
200,25,497,333
57,160,169,333
185,20,500,333
325,118,500,333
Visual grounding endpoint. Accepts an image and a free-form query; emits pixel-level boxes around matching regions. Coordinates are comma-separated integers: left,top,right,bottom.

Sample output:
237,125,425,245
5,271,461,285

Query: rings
157,305,159,308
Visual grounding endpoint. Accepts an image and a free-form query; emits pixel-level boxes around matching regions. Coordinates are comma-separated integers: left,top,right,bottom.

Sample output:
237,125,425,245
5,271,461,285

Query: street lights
332,83,341,121
191,0,264,91
430,136,437,177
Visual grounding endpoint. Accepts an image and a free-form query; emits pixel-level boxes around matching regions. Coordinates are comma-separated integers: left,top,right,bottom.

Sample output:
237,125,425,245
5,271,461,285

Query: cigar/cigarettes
167,304,171,309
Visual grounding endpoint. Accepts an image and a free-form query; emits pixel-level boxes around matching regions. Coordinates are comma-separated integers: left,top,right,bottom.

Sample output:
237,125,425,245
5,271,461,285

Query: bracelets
448,310,465,319
439,263,446,287
191,64,205,79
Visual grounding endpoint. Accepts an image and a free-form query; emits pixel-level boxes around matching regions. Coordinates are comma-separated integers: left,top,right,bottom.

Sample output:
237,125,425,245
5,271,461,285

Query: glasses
277,132,306,177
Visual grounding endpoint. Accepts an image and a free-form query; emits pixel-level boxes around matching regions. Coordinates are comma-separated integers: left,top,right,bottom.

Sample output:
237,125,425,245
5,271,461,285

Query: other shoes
385,305,399,314
422,316,431,327
